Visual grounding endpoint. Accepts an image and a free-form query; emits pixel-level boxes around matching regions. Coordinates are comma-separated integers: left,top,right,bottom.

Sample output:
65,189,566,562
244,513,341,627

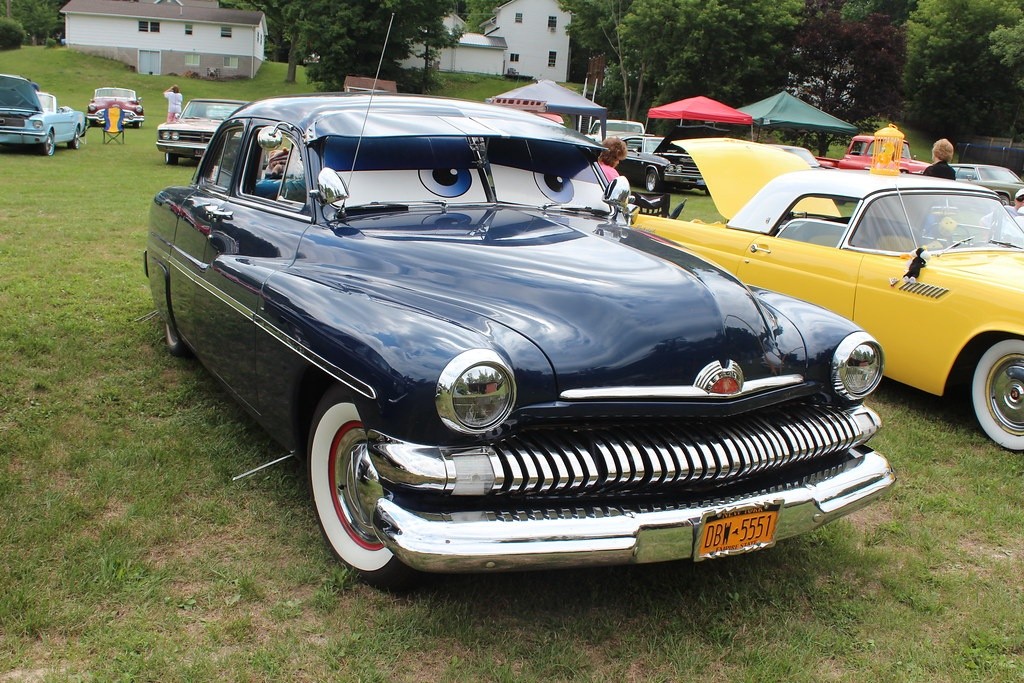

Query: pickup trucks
815,135,935,175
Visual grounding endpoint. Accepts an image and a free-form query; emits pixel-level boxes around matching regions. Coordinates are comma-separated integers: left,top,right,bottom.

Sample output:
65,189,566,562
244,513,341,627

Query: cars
155,98,252,165
630,139,1024,455
0,74,87,156
86,87,144,130
949,164,1024,205
142,96,895,590
615,136,712,196
585,119,645,145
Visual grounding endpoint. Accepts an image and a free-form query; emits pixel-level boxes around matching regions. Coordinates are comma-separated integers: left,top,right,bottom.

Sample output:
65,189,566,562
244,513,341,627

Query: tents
646,95,752,142
484,79,608,141
737,91,859,143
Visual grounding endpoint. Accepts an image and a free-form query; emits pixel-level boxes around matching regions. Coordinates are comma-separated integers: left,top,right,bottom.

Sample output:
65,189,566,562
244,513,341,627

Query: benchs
256,180,306,203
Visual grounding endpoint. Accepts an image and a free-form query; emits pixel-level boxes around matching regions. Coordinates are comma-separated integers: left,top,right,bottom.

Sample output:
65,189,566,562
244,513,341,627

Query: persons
924,139,956,180
979,187,1024,227
597,138,626,184
876,196,943,251
163,84,181,123
27,78,39,92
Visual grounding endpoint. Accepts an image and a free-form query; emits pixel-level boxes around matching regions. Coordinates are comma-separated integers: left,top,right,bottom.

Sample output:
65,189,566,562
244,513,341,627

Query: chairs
101,107,125,144
811,235,840,247
631,191,671,217
878,236,920,253
